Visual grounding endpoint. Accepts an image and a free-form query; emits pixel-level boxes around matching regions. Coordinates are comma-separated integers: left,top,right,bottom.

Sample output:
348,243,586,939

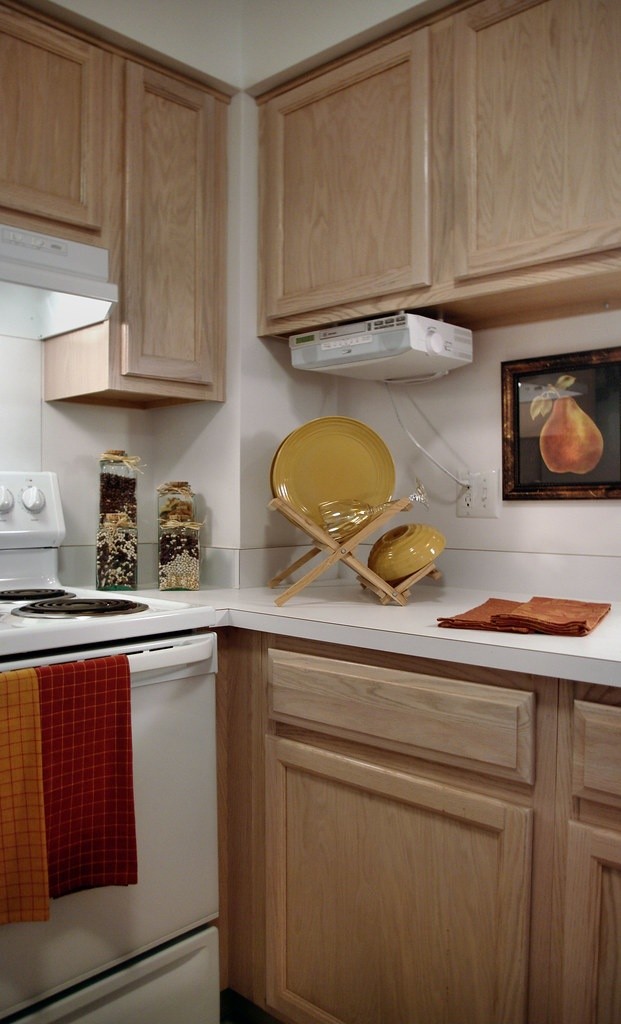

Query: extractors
0,224,118,341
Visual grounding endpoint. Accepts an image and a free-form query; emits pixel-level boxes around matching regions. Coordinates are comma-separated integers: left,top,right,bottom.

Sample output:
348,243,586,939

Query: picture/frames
500,344,621,502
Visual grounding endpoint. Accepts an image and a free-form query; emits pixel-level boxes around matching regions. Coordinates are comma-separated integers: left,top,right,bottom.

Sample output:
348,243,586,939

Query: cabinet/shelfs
253,637,559,1024
244,0,621,344
547,679,621,1024
0,0,244,410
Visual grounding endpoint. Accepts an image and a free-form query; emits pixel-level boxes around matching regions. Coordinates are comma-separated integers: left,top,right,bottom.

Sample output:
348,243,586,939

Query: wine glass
318,477,430,543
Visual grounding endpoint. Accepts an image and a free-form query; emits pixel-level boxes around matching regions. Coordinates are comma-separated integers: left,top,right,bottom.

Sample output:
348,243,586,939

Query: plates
269,428,297,498
273,416,396,526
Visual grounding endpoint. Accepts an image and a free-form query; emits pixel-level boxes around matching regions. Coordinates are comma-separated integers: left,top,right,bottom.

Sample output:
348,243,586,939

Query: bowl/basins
368,523,446,581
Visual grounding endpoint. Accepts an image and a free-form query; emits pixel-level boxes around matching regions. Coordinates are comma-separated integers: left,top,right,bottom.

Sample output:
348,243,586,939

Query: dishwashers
0,633,218,1024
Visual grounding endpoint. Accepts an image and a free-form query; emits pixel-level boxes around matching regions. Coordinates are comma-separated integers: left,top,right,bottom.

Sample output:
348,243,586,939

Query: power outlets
455,470,500,519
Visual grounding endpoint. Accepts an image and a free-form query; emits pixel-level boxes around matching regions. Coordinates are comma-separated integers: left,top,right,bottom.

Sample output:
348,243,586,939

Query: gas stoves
0,471,216,657
